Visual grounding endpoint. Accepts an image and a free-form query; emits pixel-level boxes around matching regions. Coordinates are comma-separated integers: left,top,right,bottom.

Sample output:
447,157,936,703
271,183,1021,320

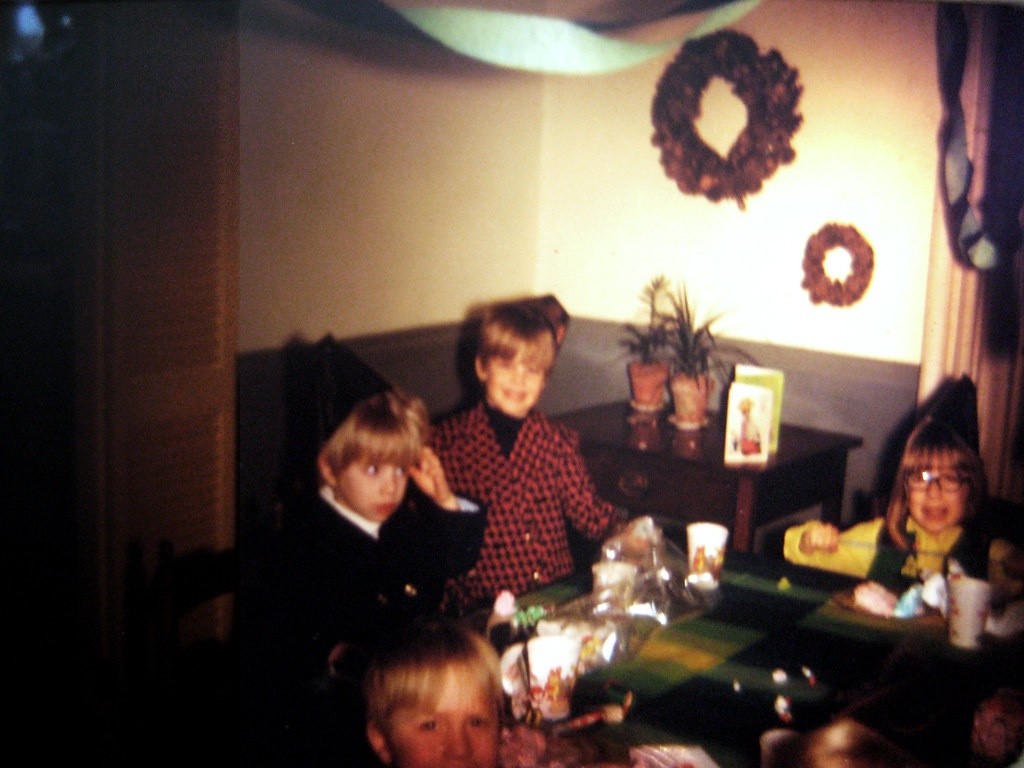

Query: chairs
120,513,263,768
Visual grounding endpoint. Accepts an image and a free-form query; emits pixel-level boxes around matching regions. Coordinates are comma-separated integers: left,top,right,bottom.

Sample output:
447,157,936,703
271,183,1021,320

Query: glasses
906,468,970,494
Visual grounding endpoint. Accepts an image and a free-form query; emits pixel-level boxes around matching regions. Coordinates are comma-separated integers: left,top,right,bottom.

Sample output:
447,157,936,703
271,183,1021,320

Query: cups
687,522,728,591
947,574,991,649
527,636,581,720
593,562,637,602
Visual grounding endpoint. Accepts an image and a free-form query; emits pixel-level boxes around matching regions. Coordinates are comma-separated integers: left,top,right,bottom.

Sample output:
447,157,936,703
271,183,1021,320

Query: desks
549,399,866,574
483,542,1024,768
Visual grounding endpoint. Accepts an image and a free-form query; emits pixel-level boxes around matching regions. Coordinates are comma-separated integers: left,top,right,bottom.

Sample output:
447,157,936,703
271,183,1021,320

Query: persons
732,397,760,455
783,374,1024,636
225,333,486,768
363,615,548,768
430,295,634,618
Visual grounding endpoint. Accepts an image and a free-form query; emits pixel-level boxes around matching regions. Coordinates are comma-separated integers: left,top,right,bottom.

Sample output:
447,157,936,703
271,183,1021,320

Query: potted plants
658,287,762,431
619,277,683,413
